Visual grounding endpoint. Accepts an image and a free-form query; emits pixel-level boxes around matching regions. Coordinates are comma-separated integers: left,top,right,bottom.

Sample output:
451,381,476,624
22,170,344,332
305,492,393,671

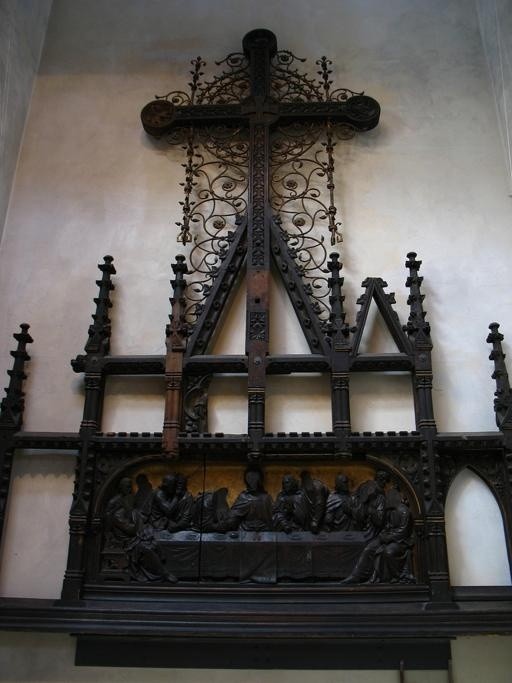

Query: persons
103,465,414,586
